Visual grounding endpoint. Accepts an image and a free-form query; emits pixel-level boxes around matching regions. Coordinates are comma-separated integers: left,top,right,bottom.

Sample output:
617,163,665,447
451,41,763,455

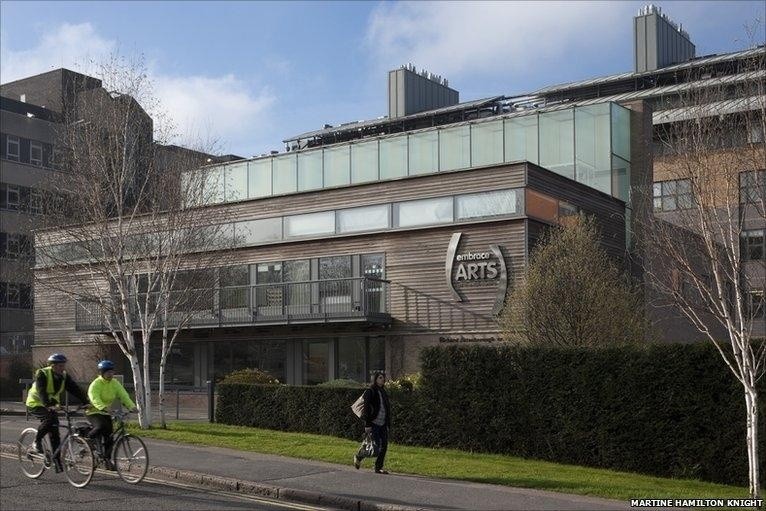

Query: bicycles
62,407,149,485
16,402,95,489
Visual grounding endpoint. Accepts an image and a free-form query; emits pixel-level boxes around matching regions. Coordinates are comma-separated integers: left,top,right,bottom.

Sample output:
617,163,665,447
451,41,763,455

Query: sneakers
31,438,44,454
54,457,63,473
375,469,389,474
103,460,116,470
354,456,360,469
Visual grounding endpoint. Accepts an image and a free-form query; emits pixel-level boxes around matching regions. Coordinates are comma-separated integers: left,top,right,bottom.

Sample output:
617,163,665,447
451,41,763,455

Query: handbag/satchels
350,387,375,418
357,433,379,458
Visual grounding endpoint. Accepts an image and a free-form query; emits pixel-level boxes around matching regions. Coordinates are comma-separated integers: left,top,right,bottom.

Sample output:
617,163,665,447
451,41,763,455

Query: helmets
97,359,116,371
48,352,67,364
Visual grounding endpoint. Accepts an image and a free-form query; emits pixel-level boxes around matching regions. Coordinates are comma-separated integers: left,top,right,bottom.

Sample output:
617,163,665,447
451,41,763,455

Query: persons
25,353,91,474
353,371,392,474
78,359,141,472
